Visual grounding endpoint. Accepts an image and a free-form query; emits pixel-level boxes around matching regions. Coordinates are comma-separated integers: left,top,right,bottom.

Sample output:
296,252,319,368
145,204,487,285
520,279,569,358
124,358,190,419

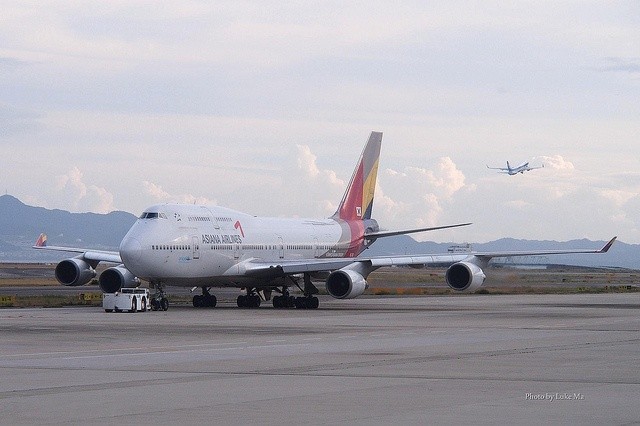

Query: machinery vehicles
102,287,151,313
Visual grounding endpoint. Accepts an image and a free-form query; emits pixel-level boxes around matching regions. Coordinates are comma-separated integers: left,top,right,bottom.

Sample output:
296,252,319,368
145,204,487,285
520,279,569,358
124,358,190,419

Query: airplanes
487,160,545,175
32,132,617,312
